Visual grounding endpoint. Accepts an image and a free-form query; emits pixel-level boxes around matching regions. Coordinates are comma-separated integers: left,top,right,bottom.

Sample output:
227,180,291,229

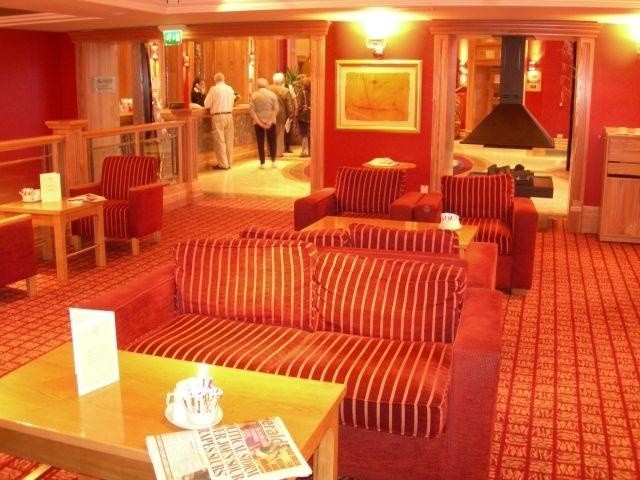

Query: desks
1,340,348,480
302,216,479,251
0,196,105,280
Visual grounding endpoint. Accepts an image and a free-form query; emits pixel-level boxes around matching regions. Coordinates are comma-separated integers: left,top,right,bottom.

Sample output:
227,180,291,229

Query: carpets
0,191,640,479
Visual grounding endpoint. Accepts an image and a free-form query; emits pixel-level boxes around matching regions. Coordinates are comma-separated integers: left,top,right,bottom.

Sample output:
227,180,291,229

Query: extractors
460,35,555,149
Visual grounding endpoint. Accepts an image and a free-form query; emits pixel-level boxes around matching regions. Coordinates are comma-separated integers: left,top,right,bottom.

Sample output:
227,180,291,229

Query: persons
249,78,280,169
191,78,206,108
203,72,235,170
284,83,297,154
269,72,292,157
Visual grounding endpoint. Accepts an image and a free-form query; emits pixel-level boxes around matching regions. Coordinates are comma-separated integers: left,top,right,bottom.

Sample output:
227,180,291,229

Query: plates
164,402,223,431
439,225,462,230
22,199,41,203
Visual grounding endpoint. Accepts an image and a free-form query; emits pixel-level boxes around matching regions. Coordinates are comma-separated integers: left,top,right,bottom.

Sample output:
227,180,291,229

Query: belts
214,112,232,115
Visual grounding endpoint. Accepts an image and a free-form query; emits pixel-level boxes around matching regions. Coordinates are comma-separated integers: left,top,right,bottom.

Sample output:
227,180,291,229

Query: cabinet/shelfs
601,126,640,243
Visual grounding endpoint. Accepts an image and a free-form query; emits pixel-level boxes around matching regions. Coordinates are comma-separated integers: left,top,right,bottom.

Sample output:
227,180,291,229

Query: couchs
238,223,500,295
294,164,425,230
416,173,538,294
1,215,39,298
71,154,169,256
84,238,504,480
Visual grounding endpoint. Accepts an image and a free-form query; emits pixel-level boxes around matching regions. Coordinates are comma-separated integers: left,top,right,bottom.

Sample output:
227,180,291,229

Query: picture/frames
334,59,423,134
